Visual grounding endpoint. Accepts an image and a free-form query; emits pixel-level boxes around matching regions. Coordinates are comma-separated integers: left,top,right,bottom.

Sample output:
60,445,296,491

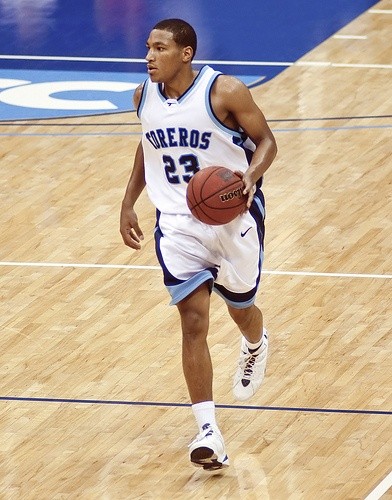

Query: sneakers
232,332,268,399
189,424,231,470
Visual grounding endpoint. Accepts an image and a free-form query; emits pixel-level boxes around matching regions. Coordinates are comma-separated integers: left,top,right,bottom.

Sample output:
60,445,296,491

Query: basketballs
183,164,249,227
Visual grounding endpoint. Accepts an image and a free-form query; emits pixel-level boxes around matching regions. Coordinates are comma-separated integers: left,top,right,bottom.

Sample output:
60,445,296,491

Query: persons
118,15,280,474
